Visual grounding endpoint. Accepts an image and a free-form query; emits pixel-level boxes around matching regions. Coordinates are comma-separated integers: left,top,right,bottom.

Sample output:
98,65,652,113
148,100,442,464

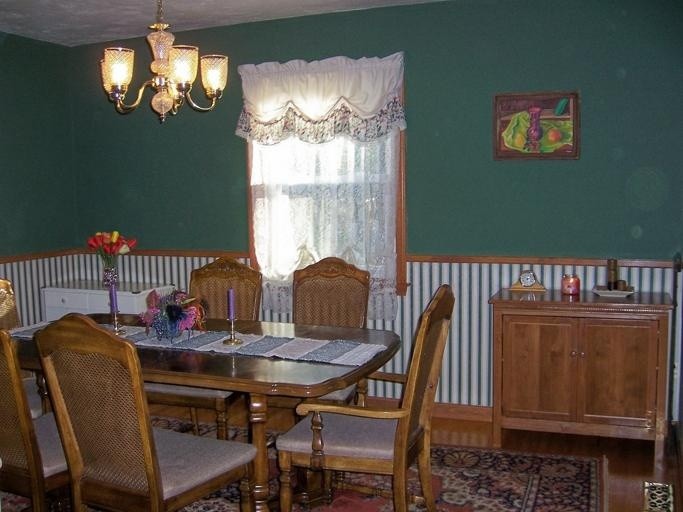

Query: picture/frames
492,92,581,160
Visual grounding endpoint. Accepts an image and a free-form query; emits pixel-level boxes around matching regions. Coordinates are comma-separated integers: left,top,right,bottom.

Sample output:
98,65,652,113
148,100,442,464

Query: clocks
506,271,548,293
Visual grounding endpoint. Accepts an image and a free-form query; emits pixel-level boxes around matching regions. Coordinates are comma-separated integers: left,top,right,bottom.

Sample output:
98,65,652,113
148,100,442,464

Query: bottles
562,274,579,296
606,258,616,291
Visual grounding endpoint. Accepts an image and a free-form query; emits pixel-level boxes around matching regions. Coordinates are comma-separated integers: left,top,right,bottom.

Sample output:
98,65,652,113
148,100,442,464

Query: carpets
0,404,615,511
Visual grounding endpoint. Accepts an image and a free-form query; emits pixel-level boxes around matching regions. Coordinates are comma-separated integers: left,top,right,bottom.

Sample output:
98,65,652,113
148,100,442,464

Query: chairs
141,254,264,440
0,277,42,422
32,312,259,511
240,254,372,495
270,283,457,510
0,328,74,512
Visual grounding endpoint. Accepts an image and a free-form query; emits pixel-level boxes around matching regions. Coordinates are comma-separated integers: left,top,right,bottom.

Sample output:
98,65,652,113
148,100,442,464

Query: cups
617,280,625,291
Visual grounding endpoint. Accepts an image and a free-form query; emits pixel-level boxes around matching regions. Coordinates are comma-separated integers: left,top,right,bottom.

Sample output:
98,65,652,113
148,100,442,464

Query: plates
591,284,634,298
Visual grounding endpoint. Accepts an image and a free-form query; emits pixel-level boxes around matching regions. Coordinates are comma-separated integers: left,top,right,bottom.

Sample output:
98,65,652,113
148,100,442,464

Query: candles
227,286,235,319
110,283,119,313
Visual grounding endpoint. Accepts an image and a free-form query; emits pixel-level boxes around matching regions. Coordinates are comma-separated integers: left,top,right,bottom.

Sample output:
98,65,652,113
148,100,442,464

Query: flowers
137,287,208,343
84,225,138,270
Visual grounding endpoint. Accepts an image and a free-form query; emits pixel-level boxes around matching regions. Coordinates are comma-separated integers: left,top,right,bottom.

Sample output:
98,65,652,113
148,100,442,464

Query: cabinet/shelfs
487,288,678,460
40,278,175,324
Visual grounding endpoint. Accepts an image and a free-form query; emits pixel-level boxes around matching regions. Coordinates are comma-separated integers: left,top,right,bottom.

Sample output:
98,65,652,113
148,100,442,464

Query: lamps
93,0,231,128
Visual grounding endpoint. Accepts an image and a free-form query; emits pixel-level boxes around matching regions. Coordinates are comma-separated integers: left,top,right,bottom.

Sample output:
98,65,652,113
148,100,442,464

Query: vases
151,314,182,342
102,267,119,288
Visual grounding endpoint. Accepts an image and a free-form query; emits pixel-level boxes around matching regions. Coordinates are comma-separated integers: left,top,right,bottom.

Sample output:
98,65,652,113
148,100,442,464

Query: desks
3,311,404,511
